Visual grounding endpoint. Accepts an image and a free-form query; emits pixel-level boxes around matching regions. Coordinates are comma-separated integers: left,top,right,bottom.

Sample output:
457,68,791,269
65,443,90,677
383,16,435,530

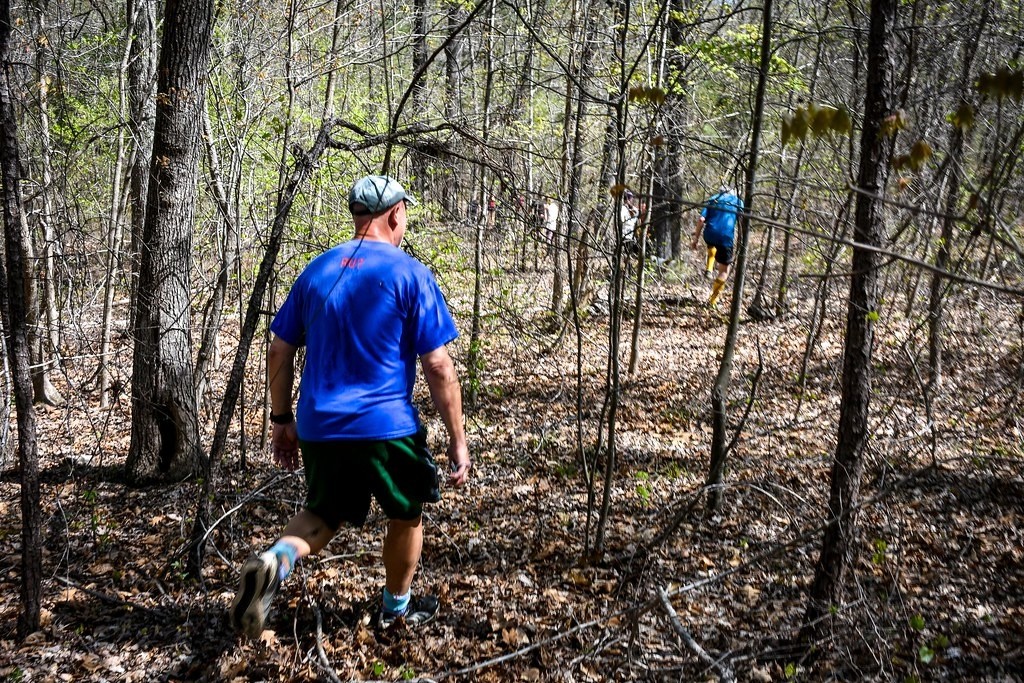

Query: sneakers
376,595,441,631
229,551,280,640
705,270,713,280
706,301,719,311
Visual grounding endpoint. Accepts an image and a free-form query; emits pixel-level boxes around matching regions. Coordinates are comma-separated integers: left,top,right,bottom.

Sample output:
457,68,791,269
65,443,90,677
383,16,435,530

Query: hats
348,174,418,216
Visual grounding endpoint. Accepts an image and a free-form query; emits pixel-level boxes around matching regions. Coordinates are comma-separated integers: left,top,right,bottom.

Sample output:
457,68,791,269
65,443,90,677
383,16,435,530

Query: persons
690,185,744,308
541,198,559,247
609,188,646,289
231,175,471,639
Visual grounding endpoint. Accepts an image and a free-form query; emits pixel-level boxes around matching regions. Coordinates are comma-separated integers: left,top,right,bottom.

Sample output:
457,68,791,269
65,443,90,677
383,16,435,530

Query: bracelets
270,410,294,425
